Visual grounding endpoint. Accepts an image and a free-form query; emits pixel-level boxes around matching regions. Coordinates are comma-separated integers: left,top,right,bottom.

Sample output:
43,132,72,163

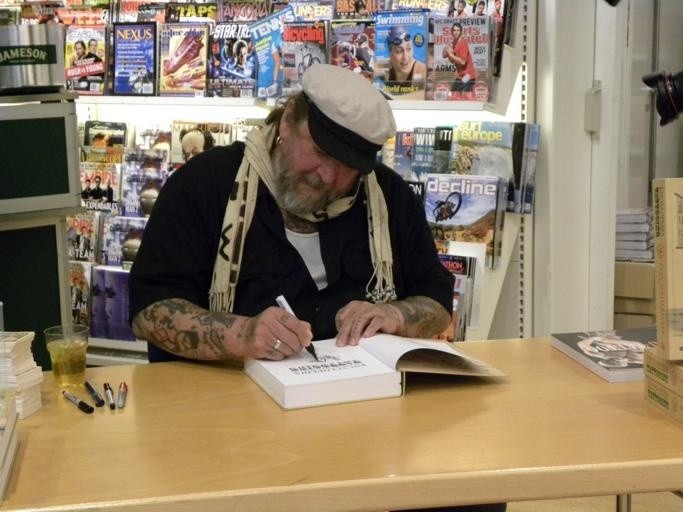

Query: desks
1,336,683,512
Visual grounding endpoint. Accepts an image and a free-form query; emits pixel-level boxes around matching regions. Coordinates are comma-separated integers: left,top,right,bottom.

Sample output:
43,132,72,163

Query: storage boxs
613,260,656,316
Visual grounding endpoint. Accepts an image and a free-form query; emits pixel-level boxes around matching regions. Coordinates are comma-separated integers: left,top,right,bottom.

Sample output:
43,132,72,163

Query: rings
274,337,281,349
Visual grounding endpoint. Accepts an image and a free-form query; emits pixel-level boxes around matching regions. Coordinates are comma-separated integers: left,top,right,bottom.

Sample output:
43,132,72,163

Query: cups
43,323,90,387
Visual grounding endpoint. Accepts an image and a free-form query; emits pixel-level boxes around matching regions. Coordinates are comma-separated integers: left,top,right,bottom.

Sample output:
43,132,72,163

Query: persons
126,64,451,363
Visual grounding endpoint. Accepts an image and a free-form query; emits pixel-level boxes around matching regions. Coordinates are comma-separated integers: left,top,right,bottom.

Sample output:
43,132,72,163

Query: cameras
640,70,683,129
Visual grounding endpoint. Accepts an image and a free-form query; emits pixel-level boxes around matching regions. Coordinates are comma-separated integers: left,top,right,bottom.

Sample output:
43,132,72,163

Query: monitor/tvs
1,216,75,373
0,102,82,220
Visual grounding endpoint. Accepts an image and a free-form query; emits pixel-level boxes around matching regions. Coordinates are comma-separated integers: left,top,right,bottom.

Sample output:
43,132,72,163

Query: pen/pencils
62,381,127,413
275,295,318,360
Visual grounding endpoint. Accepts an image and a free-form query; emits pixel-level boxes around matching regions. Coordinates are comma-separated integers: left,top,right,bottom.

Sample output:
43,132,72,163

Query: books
0,329,42,501
545,325,665,381
47,2,538,339
615,206,659,260
242,333,506,410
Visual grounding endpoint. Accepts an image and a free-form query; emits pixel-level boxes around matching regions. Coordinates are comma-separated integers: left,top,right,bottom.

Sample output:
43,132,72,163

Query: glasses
389,34,410,45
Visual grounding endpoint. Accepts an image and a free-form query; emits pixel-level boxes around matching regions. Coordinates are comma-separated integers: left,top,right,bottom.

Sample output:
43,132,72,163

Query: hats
387,24,411,48
300,62,396,174
